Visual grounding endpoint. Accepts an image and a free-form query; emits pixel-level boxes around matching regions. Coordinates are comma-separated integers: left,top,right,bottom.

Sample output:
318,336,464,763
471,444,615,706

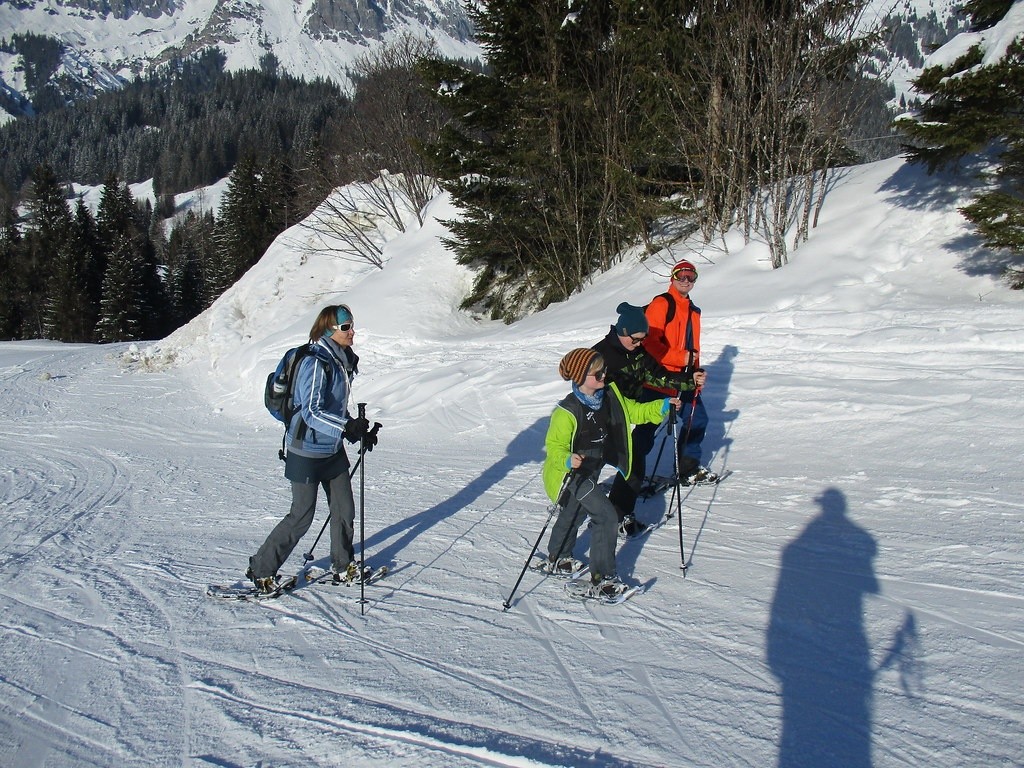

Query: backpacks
264,343,332,427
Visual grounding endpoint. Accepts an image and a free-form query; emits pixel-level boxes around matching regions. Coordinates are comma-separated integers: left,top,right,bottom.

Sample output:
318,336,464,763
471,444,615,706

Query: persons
588,302,707,539
631,259,720,497
245,304,377,594
542,348,681,598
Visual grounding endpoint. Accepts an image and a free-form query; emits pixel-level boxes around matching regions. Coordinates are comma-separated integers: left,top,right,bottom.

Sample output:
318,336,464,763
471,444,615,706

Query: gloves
360,433,378,452
346,417,369,438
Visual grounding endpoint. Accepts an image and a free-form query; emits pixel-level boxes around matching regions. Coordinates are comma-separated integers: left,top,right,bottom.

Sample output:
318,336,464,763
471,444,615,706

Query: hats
558,348,601,387
616,302,648,336
671,259,698,277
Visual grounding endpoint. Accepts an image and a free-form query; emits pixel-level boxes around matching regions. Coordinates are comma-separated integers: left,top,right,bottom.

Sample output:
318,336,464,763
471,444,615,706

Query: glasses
674,274,696,282
587,365,607,381
629,335,645,344
332,322,354,332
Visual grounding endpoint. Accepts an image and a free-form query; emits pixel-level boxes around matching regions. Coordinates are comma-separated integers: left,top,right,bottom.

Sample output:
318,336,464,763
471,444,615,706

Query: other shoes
589,577,628,598
548,556,584,576
618,519,643,538
244,566,280,593
329,561,371,583
682,469,716,484
